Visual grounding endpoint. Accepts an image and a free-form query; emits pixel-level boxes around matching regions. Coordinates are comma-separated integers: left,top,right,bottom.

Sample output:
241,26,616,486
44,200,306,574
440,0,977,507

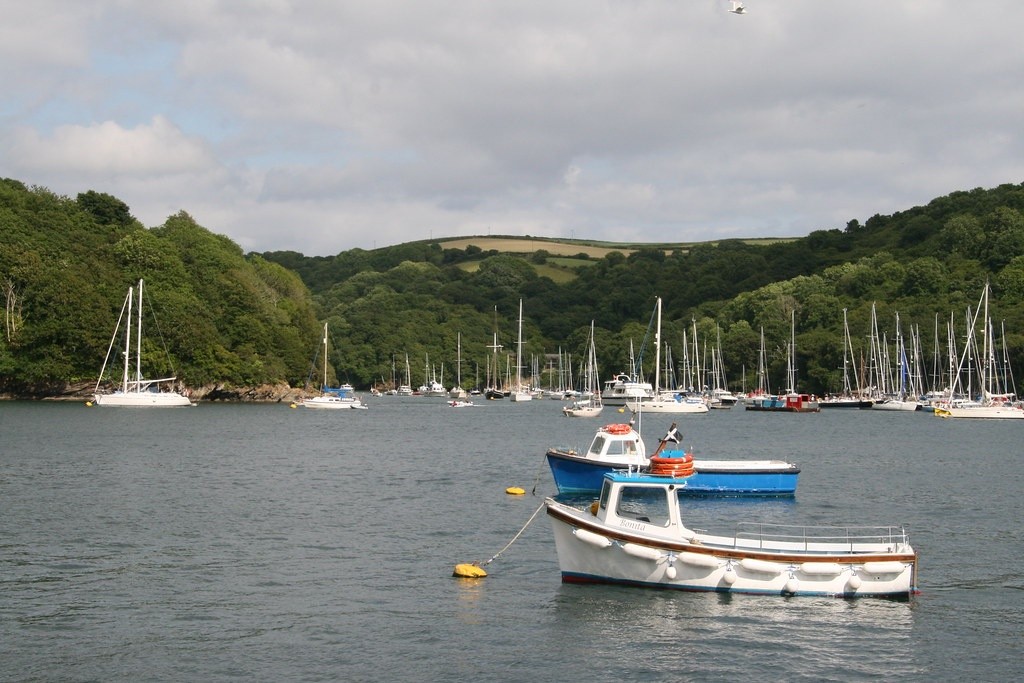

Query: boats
543,420,801,504
536,430,919,603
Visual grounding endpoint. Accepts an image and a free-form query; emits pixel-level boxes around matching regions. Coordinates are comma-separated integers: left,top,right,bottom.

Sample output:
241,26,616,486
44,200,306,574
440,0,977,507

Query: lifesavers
604,423,630,435
648,453,696,476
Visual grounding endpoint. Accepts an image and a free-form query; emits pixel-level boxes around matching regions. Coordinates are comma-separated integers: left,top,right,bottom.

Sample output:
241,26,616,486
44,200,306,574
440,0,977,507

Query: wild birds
727,0,748,14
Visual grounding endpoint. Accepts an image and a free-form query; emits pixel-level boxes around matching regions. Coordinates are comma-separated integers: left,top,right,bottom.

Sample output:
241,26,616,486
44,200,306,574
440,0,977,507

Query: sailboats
371,295,1023,420
289,322,369,410
85,277,197,407
